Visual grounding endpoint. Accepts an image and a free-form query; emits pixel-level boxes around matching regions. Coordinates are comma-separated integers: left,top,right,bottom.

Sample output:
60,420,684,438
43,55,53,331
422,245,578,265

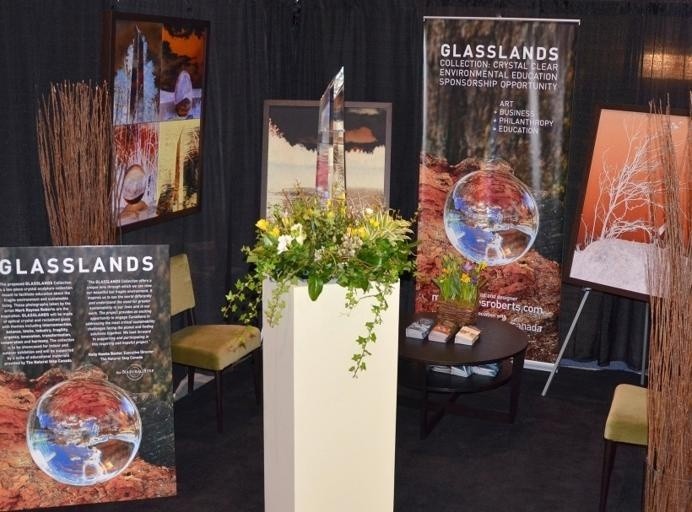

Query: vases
258,278,402,512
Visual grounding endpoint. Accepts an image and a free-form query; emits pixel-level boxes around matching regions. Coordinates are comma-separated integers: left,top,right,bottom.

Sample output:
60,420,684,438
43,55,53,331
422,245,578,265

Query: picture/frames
258,98,394,221
561,102,692,303
103,10,211,237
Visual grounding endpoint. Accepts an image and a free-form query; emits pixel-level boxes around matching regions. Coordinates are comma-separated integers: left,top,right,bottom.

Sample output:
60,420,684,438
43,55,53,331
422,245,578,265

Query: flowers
220,180,425,380
433,251,490,304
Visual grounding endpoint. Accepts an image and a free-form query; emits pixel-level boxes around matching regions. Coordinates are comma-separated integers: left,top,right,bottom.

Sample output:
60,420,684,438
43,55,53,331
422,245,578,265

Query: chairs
599,382,648,511
168,252,261,434
